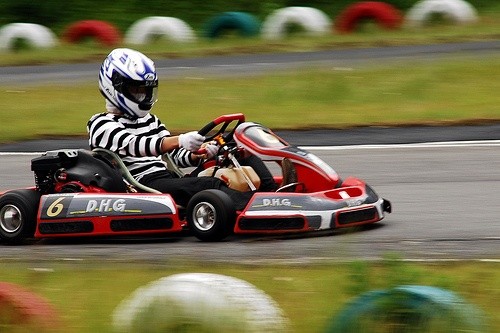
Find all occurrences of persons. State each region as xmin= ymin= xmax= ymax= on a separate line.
xmin=86 ymin=48 xmax=298 ymax=209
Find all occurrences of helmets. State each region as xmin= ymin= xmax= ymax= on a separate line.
xmin=98 ymin=48 xmax=159 ymax=121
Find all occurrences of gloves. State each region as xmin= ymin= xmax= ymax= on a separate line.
xmin=205 ymin=144 xmax=219 ymax=157
xmin=178 ymin=131 xmax=206 ymax=152
